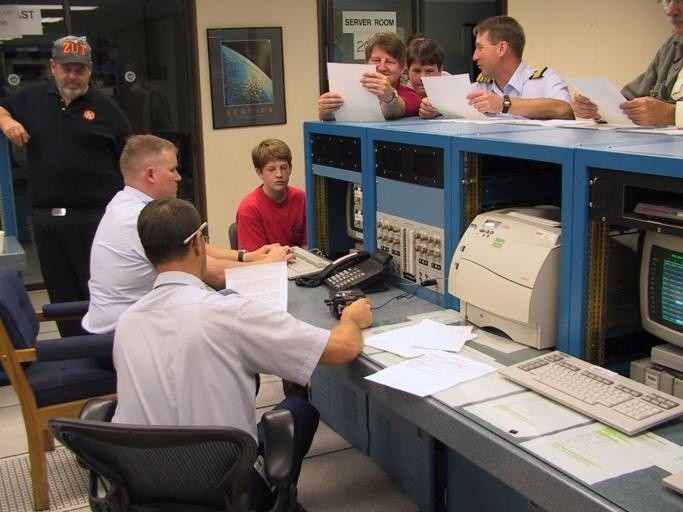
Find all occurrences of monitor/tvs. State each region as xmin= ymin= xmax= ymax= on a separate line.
xmin=346 ymin=183 xmax=363 ymax=241
xmin=639 ymin=229 xmax=682 ymax=373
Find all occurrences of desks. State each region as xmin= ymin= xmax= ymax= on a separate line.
xmin=289 ymin=241 xmax=682 ymax=512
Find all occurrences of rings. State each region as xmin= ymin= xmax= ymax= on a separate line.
xmin=374 ymin=84 xmax=377 ymax=88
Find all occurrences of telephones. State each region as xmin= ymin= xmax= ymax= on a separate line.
xmin=296 ymin=249 xmax=394 ymax=292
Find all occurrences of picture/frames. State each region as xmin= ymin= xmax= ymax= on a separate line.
xmin=206 ymin=25 xmax=286 ymax=129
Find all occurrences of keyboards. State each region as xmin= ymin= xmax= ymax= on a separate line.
xmin=287 ymin=246 xmax=332 ymax=280
xmin=498 ymin=350 xmax=682 ymax=438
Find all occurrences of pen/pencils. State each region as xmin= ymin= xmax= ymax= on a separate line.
xmin=184 ymin=222 xmax=208 ymax=244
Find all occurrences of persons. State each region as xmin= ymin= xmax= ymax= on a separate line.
xmin=112 ymin=197 xmax=375 ymax=512
xmin=235 ymin=139 xmax=309 ymax=253
xmin=79 ymin=134 xmax=299 ymax=399
xmin=317 ymin=32 xmax=422 ymax=120
xmin=419 ymin=16 xmax=575 ymax=122
xmin=0 ymin=35 xmax=139 ymax=339
xmin=401 ymin=36 xmax=454 ymax=96
xmin=571 ymin=0 xmax=683 ymax=129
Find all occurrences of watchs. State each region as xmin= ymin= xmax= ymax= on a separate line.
xmin=383 ymin=88 xmax=398 ymax=104
xmin=502 ymin=95 xmax=512 ymax=113
xmin=237 ymin=249 xmax=247 ymax=262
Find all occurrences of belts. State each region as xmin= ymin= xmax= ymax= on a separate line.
xmin=31 ymin=205 xmax=105 ymax=216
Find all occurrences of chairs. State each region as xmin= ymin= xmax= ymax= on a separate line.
xmin=47 ymin=417 xmax=282 ymax=509
xmin=0 ymin=262 xmax=118 ymax=511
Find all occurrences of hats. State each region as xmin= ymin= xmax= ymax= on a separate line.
xmin=52 ymin=36 xmax=92 ymax=67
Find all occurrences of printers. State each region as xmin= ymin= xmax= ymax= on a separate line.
xmin=447 ymin=202 xmax=561 ymax=349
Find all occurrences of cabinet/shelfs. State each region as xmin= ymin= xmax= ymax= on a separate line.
xmin=303 ymin=116 xmax=683 ymax=359
